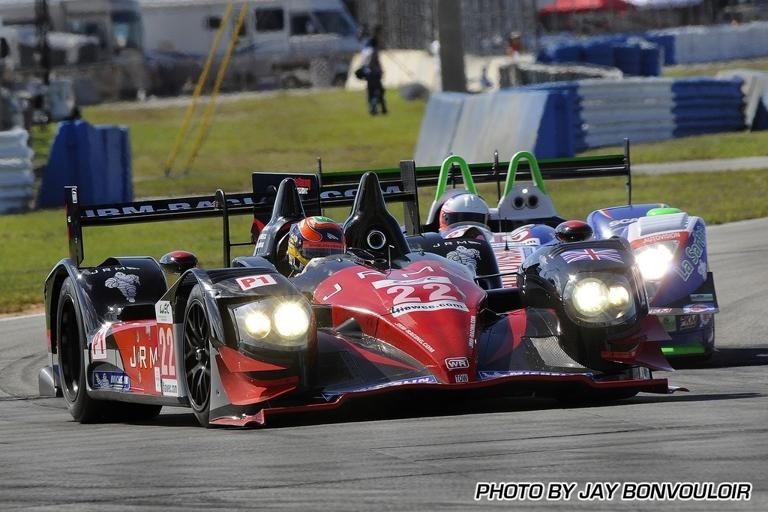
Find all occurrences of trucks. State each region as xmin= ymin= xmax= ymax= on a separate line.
xmin=0 ymin=1 xmax=363 ymax=88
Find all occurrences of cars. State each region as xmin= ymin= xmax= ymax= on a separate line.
xmin=33 ymin=158 xmax=692 ymax=430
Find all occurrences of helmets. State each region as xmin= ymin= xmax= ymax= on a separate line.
xmin=286 ymin=215 xmax=347 ymax=270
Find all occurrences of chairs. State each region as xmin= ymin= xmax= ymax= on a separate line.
xmin=238 ymin=172 xmax=413 ymax=272
xmin=417 ymin=151 xmax=570 ymax=233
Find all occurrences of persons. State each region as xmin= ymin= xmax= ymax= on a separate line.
xmin=283 ymin=214 xmax=346 ymax=278
xmin=353 ymin=24 xmax=390 ymax=116
